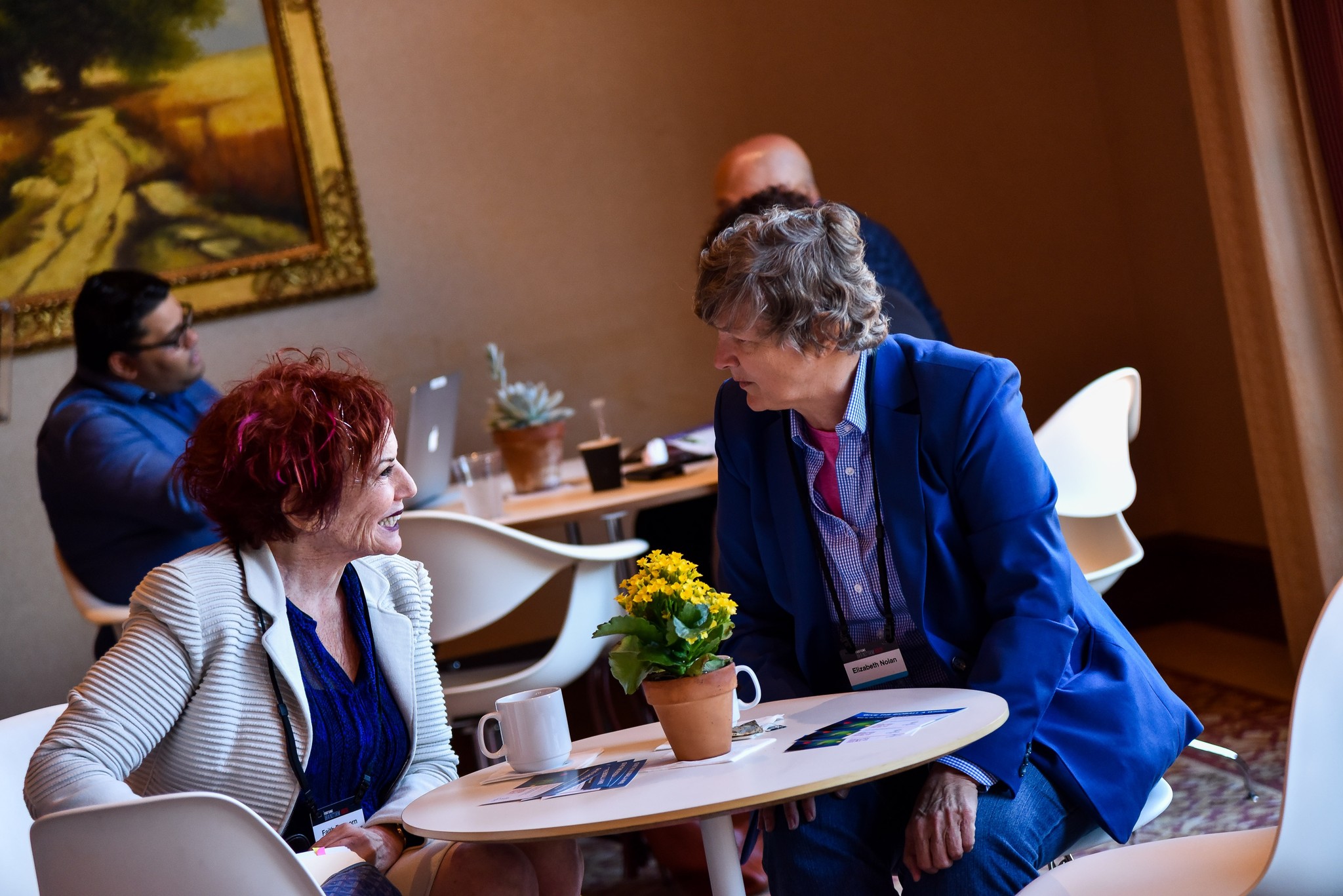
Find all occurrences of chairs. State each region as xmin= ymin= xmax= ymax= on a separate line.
xmin=1 ymin=509 xmax=650 ymax=896
xmin=1014 ymin=368 xmax=1343 ymax=896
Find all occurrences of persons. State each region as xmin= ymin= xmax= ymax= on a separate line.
xmin=692 ymin=200 xmax=1206 ymax=896
xmin=34 ymin=267 xmax=227 ymax=661
xmin=699 ymin=134 xmax=956 ymax=349
xmin=23 ymin=347 xmax=583 ymax=895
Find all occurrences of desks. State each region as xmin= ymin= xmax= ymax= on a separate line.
xmin=422 ymin=456 xmax=719 ymax=577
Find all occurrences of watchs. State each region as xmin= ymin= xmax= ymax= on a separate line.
xmin=392 ymin=823 xmax=409 ymax=846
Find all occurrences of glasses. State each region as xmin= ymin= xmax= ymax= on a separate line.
xmin=125 ymin=300 xmax=193 ymax=353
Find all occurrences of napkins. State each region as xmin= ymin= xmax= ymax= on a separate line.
xmin=639 ymin=714 xmax=786 ymax=773
xmin=480 ymin=748 xmax=603 ymax=788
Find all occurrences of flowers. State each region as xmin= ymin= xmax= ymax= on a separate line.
xmin=588 ymin=541 xmax=739 ymax=701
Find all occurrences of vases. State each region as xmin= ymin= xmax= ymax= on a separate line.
xmin=640 ymin=657 xmax=738 ymax=764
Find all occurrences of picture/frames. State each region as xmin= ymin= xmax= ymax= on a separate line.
xmin=0 ymin=0 xmax=379 ymax=358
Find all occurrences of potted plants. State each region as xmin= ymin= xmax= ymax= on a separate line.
xmin=481 ymin=340 xmax=579 ymax=493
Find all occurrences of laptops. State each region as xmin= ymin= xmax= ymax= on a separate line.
xmin=401 ymin=374 xmax=461 ymax=509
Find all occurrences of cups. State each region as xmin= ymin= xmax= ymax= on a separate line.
xmin=697 ymin=656 xmax=761 ymax=727
xmin=577 ymin=437 xmax=622 ymax=492
xmin=451 ymin=448 xmax=502 ymax=519
xmin=477 ymin=688 xmax=572 ymax=773
